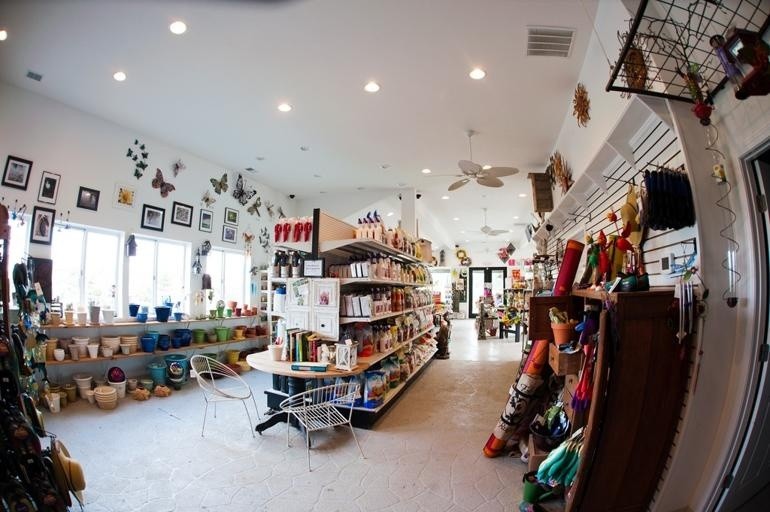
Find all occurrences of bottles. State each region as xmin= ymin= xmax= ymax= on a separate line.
xmin=391 ymin=286 xmax=406 ymax=311
xmin=394 ymin=232 xmax=416 ymax=257
xmin=389 ymin=324 xmax=422 ymax=344
xmin=391 ymin=262 xmax=414 ymax=283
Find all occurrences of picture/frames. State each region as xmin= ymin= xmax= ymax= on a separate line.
xmin=0 ymin=155 xmax=239 ymax=245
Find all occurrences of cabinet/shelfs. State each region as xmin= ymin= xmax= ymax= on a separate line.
xmin=497 ymin=288 xmax=533 ymax=341
xmin=529 ymin=289 xmax=697 ymax=512
xmin=246 ymin=208 xmax=438 ymax=430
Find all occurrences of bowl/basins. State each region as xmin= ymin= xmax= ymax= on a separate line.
xmin=33 ymin=302 xmax=267 ymax=413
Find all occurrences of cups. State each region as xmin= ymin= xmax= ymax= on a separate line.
xmin=266 ymin=345 xmax=286 ymax=362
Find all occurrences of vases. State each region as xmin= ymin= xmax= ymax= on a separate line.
xmin=30 ymin=301 xmax=270 ymax=410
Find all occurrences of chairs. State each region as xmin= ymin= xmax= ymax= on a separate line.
xmin=279 ymin=382 xmax=366 ymax=472
xmin=191 ymin=355 xmax=260 ymax=438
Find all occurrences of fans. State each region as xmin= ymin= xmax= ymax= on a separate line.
xmin=448 ymin=130 xmax=519 ymax=191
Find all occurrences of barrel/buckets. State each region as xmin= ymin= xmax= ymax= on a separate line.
xmin=550 ymin=320 xmax=579 ymax=346
xmin=31 ymin=300 xmax=268 ymax=414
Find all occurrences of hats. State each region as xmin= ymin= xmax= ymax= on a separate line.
xmin=621 ymin=183 xmax=643 ymax=246
xmin=52 ymin=440 xmax=85 ymax=508
